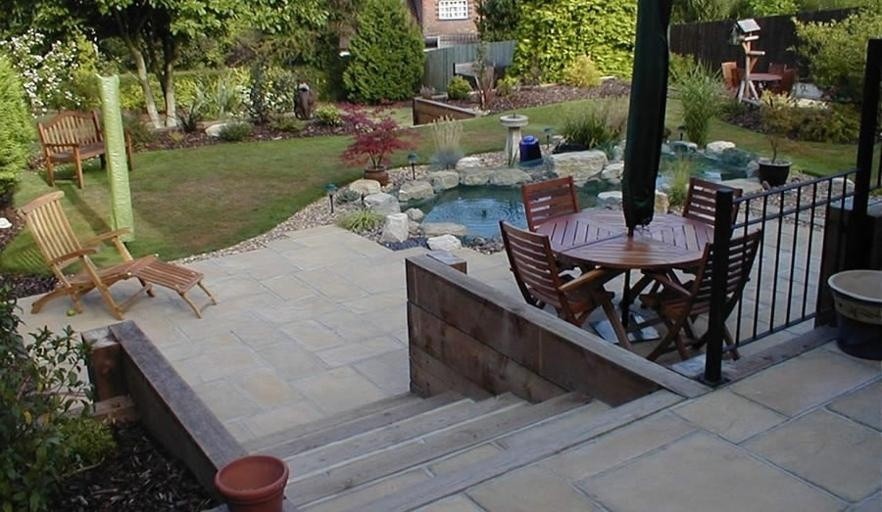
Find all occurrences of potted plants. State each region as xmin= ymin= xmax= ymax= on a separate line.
xmin=757 ymin=95 xmax=805 ymax=191
xmin=334 ymin=97 xmax=425 ymax=187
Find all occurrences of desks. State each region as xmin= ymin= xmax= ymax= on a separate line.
xmin=536 ymin=209 xmax=719 ymax=346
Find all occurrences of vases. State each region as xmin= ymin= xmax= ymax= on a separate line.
xmin=214 ymin=453 xmax=289 ymax=512
xmin=828 ymin=269 xmax=882 ymax=361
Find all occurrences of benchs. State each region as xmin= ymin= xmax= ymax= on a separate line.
xmin=37 ymin=109 xmax=136 ymax=189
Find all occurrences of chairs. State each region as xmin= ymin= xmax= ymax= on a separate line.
xmin=522 ymin=174 xmax=616 ymax=310
xmin=499 ymin=219 xmax=635 ymax=352
xmin=638 ymin=228 xmax=762 ymax=362
xmin=640 ymin=175 xmax=743 ymax=309
xmin=722 ymin=61 xmax=798 ymax=98
xmin=20 ymin=190 xmax=220 ymax=320
